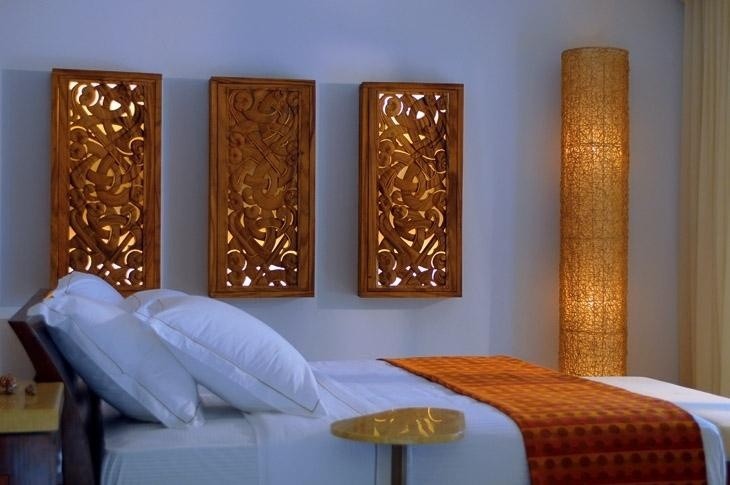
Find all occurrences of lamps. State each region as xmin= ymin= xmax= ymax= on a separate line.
xmin=558 ymin=46 xmax=631 ymax=382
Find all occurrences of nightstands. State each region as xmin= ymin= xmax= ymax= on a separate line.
xmin=0 ymin=378 xmax=67 ymax=485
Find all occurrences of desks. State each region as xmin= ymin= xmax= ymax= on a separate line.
xmin=331 ymin=407 xmax=465 ymax=485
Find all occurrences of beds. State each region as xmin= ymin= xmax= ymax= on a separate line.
xmin=6 ymin=288 xmax=729 ymax=484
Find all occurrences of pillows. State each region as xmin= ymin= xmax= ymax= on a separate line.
xmin=117 ymin=289 xmax=330 ymax=422
xmin=25 ymin=270 xmax=205 ymax=432
